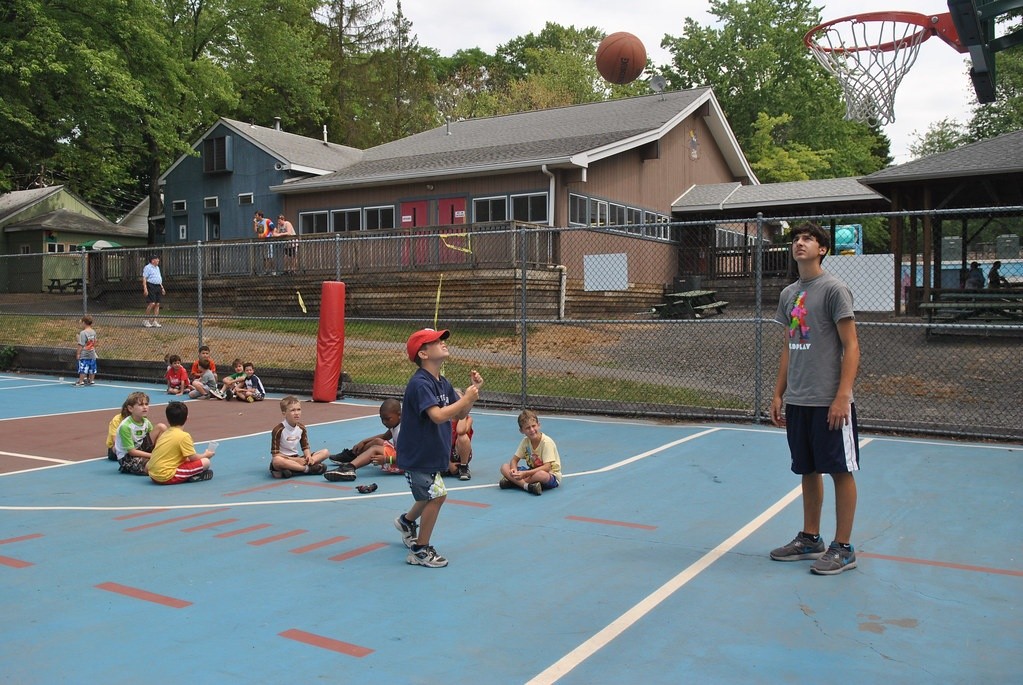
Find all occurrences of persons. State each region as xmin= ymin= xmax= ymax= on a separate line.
xmin=499 ymin=410 xmax=562 ymax=496
xmin=440 ymin=387 xmax=473 ymax=480
xmin=106 ymin=400 xmax=132 ymax=462
xmin=140 ymin=254 xmax=165 ymax=328
xmin=164 ymin=346 xmax=266 ymax=403
xmin=768 ymin=220 xmax=860 ymax=575
xmin=146 ymin=401 xmax=215 ymax=485
xmin=269 ymin=396 xmax=330 ymax=479
xmin=967 ymin=262 xmax=984 ymax=290
xmin=272 ymin=214 xmax=298 ymax=275
xmin=115 ymin=391 xmax=168 ymax=476
xmin=987 ymin=261 xmax=1005 ymax=290
xmin=323 ymin=398 xmax=406 ymax=482
xmin=394 ymin=328 xmax=483 ymax=569
xmin=70 ymin=315 xmax=97 ymax=387
xmin=252 ymin=209 xmax=277 ymax=275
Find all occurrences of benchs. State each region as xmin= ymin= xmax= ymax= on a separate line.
xmin=45 ymin=283 xmax=84 ymax=289
xmin=693 ymin=300 xmax=730 ymax=310
xmin=922 ymin=315 xmax=1023 ymax=340
xmin=650 ymin=299 xmax=684 ymax=308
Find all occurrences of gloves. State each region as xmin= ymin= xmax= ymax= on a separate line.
xmin=356 ymin=483 xmax=378 ymax=493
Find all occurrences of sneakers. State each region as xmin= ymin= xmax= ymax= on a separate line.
xmin=528 ymin=482 xmax=542 ymax=495
xmin=305 ymin=463 xmax=327 ymax=476
xmin=407 ymin=543 xmax=448 ymax=567
xmin=226 ymin=388 xmax=234 ymax=402
xmin=142 ymin=320 xmax=161 ymax=327
xmin=272 ymin=470 xmax=294 ymax=478
xmin=395 ymin=513 xmax=420 ymax=548
xmin=499 ymin=477 xmax=516 ymax=489
xmin=236 ymin=396 xmax=243 ymax=402
xmin=810 ymin=541 xmax=857 ymax=574
xmin=459 ymin=463 xmax=471 ymax=479
xmin=324 ymin=464 xmax=356 ymax=482
xmin=197 ymin=394 xmax=210 ymax=400
xmin=771 ymin=531 xmax=827 ymax=561
xmin=328 ymin=448 xmax=357 ymax=464
xmin=247 ymin=396 xmax=253 ymax=403
xmin=210 ymin=388 xmax=224 ymax=399
xmin=188 ymin=470 xmax=214 ymax=482
xmin=76 ymin=380 xmax=95 ymax=388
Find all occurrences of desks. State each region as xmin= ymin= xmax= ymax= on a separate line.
xmin=662 ymin=290 xmax=723 ymax=320
xmin=939 ymin=292 xmax=1023 ymax=301
xmin=47 ymin=278 xmax=84 ymax=294
xmin=919 ymin=301 xmax=1023 ymax=318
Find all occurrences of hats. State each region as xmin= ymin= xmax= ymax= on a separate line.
xmin=406 ymin=328 xmax=450 ymax=361
xmin=149 ymin=255 xmax=158 ymax=259
xmin=971 ymin=262 xmax=981 ymax=267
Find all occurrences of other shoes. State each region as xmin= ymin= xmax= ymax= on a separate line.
xmin=256 ymin=271 xmax=277 ymax=276
xmin=183 ymin=389 xmax=190 ymax=395
xmin=290 ymin=269 xmax=296 ymax=276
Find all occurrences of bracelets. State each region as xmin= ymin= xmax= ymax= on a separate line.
xmin=390 ymin=456 xmax=392 ymax=465
xmin=384 ymin=455 xmax=387 ymax=464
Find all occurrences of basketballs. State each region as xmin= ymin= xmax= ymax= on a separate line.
xmin=596 ymin=31 xmax=648 ymax=85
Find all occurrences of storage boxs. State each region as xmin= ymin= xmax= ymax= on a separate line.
xmin=674 ymin=277 xmax=701 ymax=290
